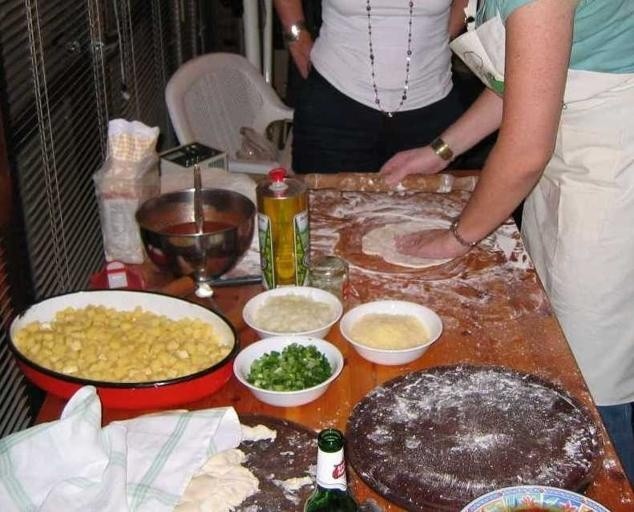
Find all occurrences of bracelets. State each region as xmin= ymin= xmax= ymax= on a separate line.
xmin=428 ymin=135 xmax=454 ymax=160
xmin=282 ymin=21 xmax=308 ymax=42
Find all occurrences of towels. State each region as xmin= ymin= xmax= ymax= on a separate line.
xmin=1 ymin=381 xmax=244 ymax=512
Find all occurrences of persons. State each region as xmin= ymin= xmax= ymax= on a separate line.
xmin=275 ymin=0 xmax=467 ymax=173
xmin=375 ymin=0 xmax=634 ymax=485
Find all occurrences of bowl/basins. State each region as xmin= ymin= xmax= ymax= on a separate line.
xmin=234 ymin=288 xmax=444 ymax=410
xmin=133 ymin=188 xmax=257 ymax=278
xmin=9 ymin=289 xmax=239 ymax=412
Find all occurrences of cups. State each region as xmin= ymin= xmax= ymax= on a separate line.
xmin=312 ymin=257 xmax=350 ymax=314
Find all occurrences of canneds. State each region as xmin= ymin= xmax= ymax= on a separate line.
xmin=308 ymin=256 xmax=349 ymax=310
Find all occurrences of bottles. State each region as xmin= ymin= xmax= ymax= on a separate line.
xmin=302 ymin=429 xmax=361 ymax=512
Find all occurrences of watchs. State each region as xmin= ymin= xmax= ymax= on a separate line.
xmin=448 ymin=219 xmax=478 ymax=247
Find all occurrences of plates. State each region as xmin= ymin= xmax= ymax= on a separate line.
xmin=460 ymin=484 xmax=611 ymax=512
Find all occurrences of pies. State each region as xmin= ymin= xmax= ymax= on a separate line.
xmin=362 ymin=219 xmax=454 ymax=269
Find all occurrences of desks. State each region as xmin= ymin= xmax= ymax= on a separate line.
xmin=23 ymin=154 xmax=634 ymax=512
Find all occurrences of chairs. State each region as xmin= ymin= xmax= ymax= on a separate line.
xmin=162 ymin=48 xmax=300 ymax=174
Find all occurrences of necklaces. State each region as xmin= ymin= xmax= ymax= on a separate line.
xmin=368 ymin=1 xmax=414 ymax=119
